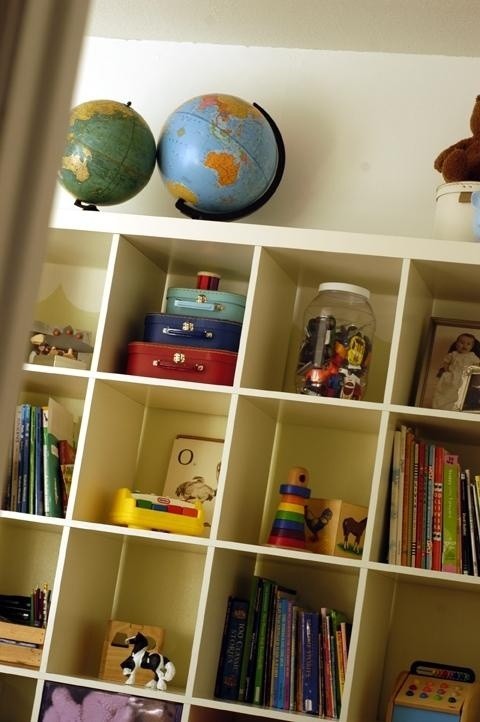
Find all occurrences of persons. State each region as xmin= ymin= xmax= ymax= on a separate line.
xmin=431 ymin=333 xmax=480 ymax=412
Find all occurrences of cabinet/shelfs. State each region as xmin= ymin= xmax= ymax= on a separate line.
xmin=0 ymin=203 xmax=480 ymax=722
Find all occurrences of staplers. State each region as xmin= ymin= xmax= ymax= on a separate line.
xmin=0 ymin=594 xmax=31 ymax=621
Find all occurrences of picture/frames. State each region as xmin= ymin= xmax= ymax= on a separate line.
xmin=407 ymin=315 xmax=480 ymax=413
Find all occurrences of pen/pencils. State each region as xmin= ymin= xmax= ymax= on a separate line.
xmin=32 ymin=582 xmax=51 ymax=629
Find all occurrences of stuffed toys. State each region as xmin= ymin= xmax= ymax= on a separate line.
xmin=434 ymin=94 xmax=480 ymax=184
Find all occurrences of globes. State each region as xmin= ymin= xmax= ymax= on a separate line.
xmin=55 ymin=100 xmax=156 ymax=211
xmin=158 ymin=94 xmax=286 ymax=223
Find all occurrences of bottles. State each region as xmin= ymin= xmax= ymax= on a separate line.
xmin=294 ymin=282 xmax=377 ymax=400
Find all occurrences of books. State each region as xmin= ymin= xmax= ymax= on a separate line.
xmin=383 ymin=424 xmax=480 ymax=577
xmin=211 ymin=574 xmax=349 ymax=720
xmin=3 ymin=397 xmax=83 ymax=518
